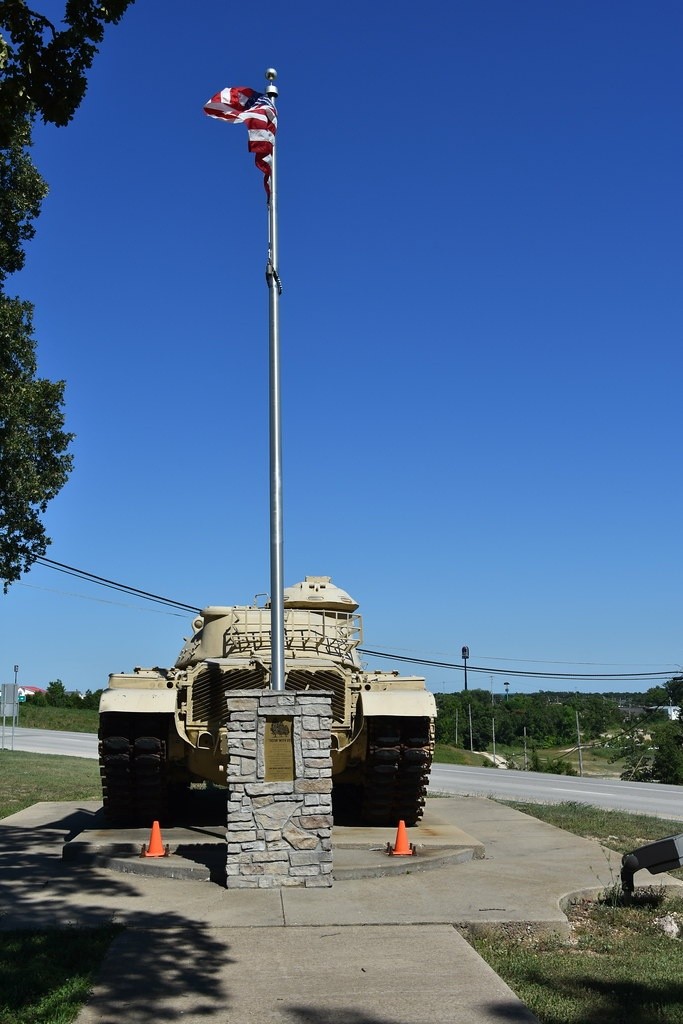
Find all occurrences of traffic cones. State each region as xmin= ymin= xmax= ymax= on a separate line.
xmin=143 ymin=820 xmax=172 ymax=859
xmin=387 ymin=820 xmax=416 ymax=856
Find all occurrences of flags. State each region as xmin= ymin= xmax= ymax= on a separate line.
xmin=203 ymin=87 xmax=277 ymax=197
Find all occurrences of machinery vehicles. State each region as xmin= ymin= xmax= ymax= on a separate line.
xmin=97 ymin=574 xmax=441 ymax=825
